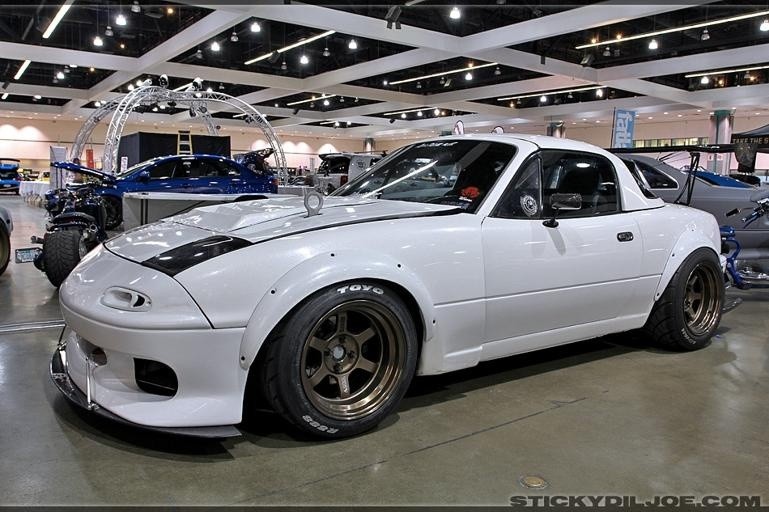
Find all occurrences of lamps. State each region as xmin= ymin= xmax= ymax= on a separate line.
xmin=648 ymin=7 xmax=769 ymax=50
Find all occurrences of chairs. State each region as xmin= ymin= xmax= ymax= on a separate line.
xmin=462 ymin=145 xmax=608 ymax=215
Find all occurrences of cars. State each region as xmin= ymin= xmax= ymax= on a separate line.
xmin=0 ymin=129 xmax=769 ymax=448
